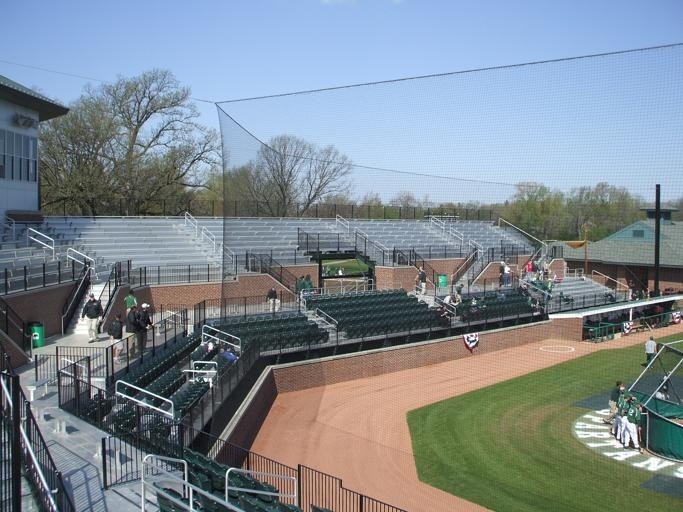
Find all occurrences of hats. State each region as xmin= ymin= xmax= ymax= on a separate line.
xmin=141 ymin=303 xmax=150 ymax=308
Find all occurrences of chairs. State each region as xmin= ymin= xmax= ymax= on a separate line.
xmin=116 ymin=426 xmax=331 ymax=512
xmin=70 ymin=333 xmax=237 ymax=431
xmin=202 ymin=310 xmax=329 ymax=352
xmin=301 ymin=288 xmax=452 ymax=340
xmin=434 ymin=287 xmax=540 ymax=324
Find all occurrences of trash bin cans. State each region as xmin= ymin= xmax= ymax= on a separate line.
xmin=28 ymin=322 xmax=45 ymax=348
xmin=437 ymin=274 xmax=447 ymax=287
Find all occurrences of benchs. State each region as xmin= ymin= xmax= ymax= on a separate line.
xmin=43 ymin=407 xmax=70 ymax=434
xmin=0 ymin=215 xmax=537 ymax=297
xmin=26 ymin=378 xmax=50 ymax=402
xmin=518 ymin=275 xmax=623 ymax=313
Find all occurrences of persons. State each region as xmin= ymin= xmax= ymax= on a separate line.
xmin=136 ymin=303 xmax=154 ymax=352
xmin=454 ymin=283 xmax=464 ymax=302
xmin=123 ymin=289 xmax=137 ymax=316
xmin=443 ymin=294 xmax=457 ymax=306
xmin=496 ymin=258 xmax=559 ymax=297
xmin=264 ymin=285 xmax=281 ymax=311
xmin=125 ymin=305 xmax=138 ymax=361
xmin=80 ymin=293 xmax=104 ymax=342
xmin=644 ymin=335 xmax=657 ymax=369
xmin=107 ymin=312 xmax=122 ymax=366
xmin=602 ymin=380 xmax=643 ymax=452
xmin=414 ymin=267 xmax=427 ymax=295
xmin=298 ymin=273 xmax=312 ymax=292
